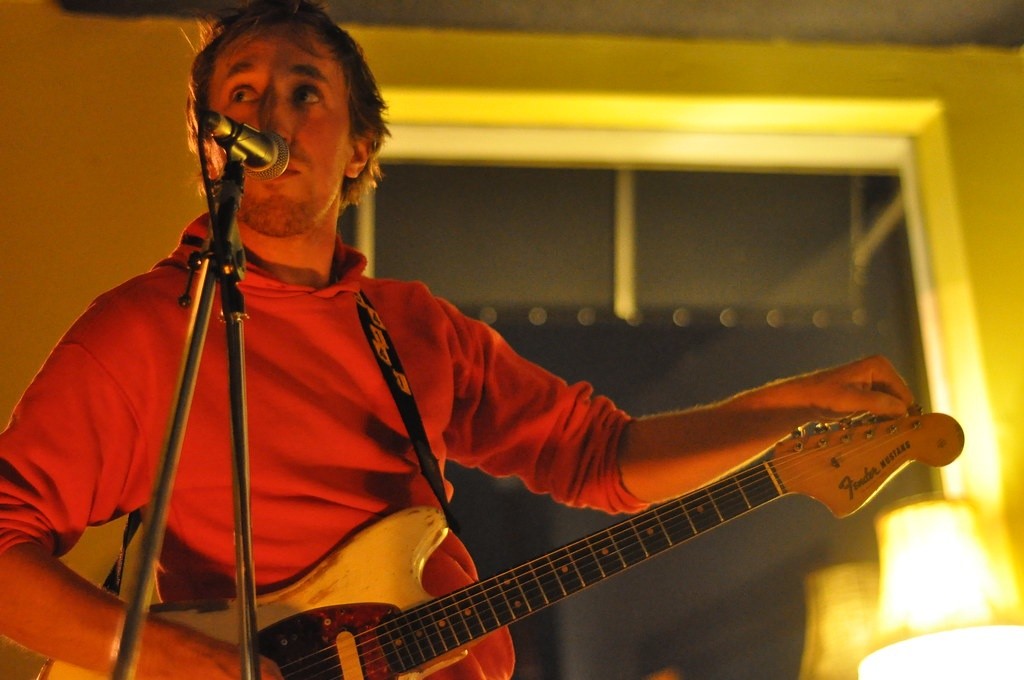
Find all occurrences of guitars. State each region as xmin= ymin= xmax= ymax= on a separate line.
xmin=26 ymin=398 xmax=966 ymax=680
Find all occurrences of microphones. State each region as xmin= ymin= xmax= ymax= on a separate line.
xmin=196 ymin=111 xmax=291 ymax=180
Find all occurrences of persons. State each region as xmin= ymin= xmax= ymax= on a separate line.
xmin=0 ymin=1 xmax=921 ymax=680
